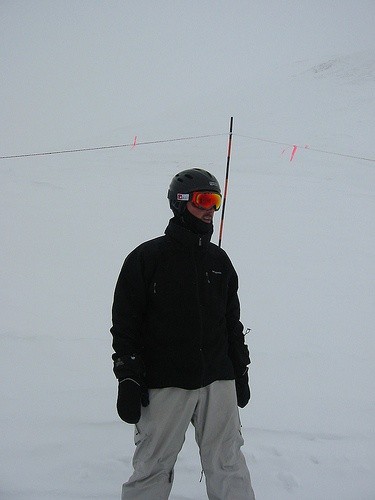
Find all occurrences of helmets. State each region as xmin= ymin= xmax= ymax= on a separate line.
xmin=167 ymin=168 xmax=222 ymax=201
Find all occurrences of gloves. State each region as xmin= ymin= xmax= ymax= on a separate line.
xmin=112 ymin=353 xmax=149 ymax=424
xmin=235 ymin=365 xmax=250 ymax=408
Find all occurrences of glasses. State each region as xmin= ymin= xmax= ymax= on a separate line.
xmin=188 ymin=191 xmax=223 ymax=212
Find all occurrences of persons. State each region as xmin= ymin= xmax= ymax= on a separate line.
xmin=110 ymin=168 xmax=256 ymax=500
